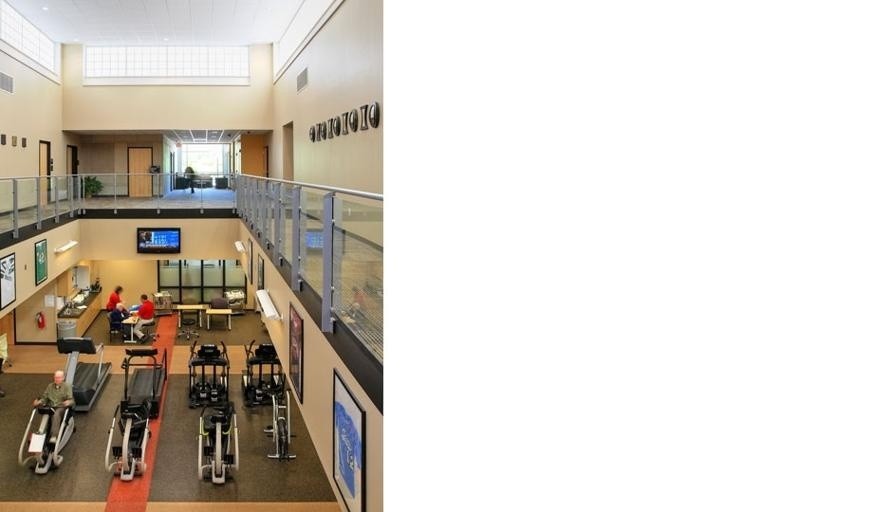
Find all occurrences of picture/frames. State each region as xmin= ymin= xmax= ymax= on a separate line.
xmin=1 ymin=252 xmax=18 ymax=309
xmin=245 ymin=238 xmax=267 ymax=293
xmin=32 ymin=237 xmax=49 ymax=285
xmin=327 ymin=367 xmax=371 ymax=512
xmin=285 ymin=302 xmax=307 ymax=404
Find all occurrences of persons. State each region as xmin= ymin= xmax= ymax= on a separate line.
xmin=139 ymin=232 xmax=152 ymax=246
xmin=111 ymin=302 xmax=130 ymax=339
xmin=32 ymin=370 xmax=76 ymax=444
xmin=107 ymin=286 xmax=122 ymax=311
xmin=133 ymin=294 xmax=154 ymax=342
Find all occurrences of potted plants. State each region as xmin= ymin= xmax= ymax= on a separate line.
xmin=83 ymin=174 xmax=102 ymax=200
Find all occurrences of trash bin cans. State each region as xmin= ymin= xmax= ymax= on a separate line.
xmin=58 ymin=320 xmax=76 ymax=337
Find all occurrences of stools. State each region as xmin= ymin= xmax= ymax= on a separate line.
xmin=178 ymin=318 xmax=199 ymax=341
xmin=138 ymin=319 xmax=156 ymax=340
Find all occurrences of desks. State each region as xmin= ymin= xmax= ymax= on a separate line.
xmin=123 ymin=308 xmax=142 ymax=344
xmin=178 ymin=299 xmax=232 ymax=332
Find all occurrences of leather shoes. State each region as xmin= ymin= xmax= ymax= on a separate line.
xmin=124 ymin=337 xmax=130 ymax=340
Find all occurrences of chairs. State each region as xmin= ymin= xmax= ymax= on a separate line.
xmin=104 ymin=309 xmax=123 ymax=345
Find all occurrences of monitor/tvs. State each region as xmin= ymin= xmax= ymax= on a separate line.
xmin=300 ymin=229 xmax=345 ymax=256
xmin=137 ymin=227 xmax=180 ymax=254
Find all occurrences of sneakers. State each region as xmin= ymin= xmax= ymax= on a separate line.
xmin=141 ymin=335 xmax=149 ymax=344
xmin=48 ymin=436 xmax=56 ymax=444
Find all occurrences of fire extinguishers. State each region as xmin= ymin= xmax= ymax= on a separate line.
xmin=36 ymin=311 xmax=44 ymax=328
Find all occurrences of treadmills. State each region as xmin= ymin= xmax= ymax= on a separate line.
xmin=120 ymin=348 xmax=167 ymax=419
xmin=57 ymin=336 xmax=111 ymax=412
